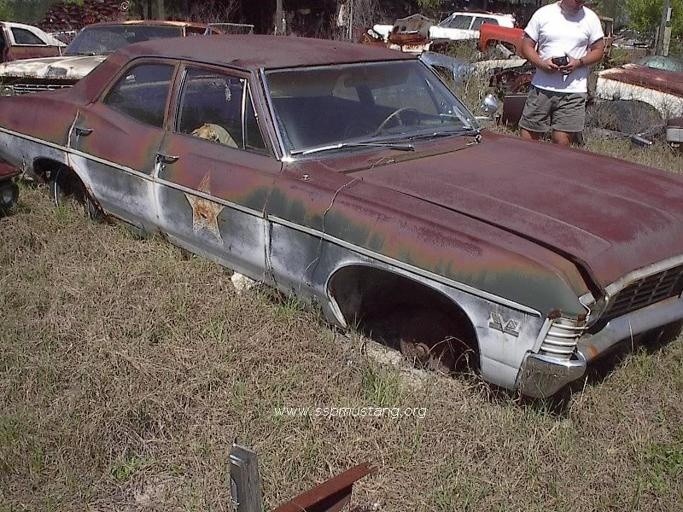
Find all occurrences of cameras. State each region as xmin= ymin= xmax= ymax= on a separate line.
xmin=552 ymin=56 xmax=568 ymax=65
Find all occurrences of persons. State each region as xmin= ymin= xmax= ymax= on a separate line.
xmin=519 ymin=0 xmax=605 ymax=147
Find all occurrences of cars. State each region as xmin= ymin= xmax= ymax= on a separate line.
xmin=0 ymin=21 xmax=231 ymax=98
xmin=0 ymin=31 xmax=682 ymax=407
xmin=369 ymin=10 xmax=518 ymax=48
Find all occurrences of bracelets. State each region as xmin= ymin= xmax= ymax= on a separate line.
xmin=580 ymin=57 xmax=583 ymax=66
